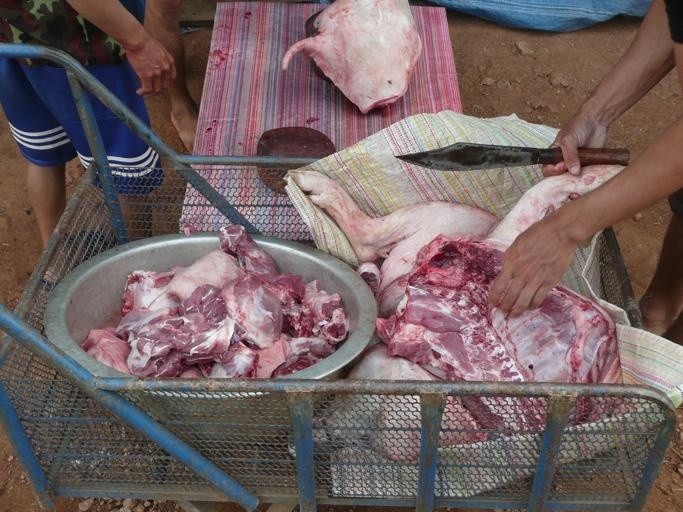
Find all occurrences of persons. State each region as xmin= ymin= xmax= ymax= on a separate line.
xmin=0 ymin=0 xmax=179 ymax=286
xmin=144 ymin=0 xmax=203 ymax=156
xmin=487 ymin=1 xmax=683 ymax=350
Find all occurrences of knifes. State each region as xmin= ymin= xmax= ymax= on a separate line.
xmin=395 ymin=139 xmax=630 ymax=172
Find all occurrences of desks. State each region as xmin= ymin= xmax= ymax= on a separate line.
xmin=179 ymin=1 xmax=463 ymax=248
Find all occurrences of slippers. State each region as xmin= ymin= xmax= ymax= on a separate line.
xmin=41 ymin=232 xmax=111 ymax=291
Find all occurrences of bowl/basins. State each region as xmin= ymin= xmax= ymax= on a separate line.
xmin=42 ymin=230 xmax=377 ymax=438
